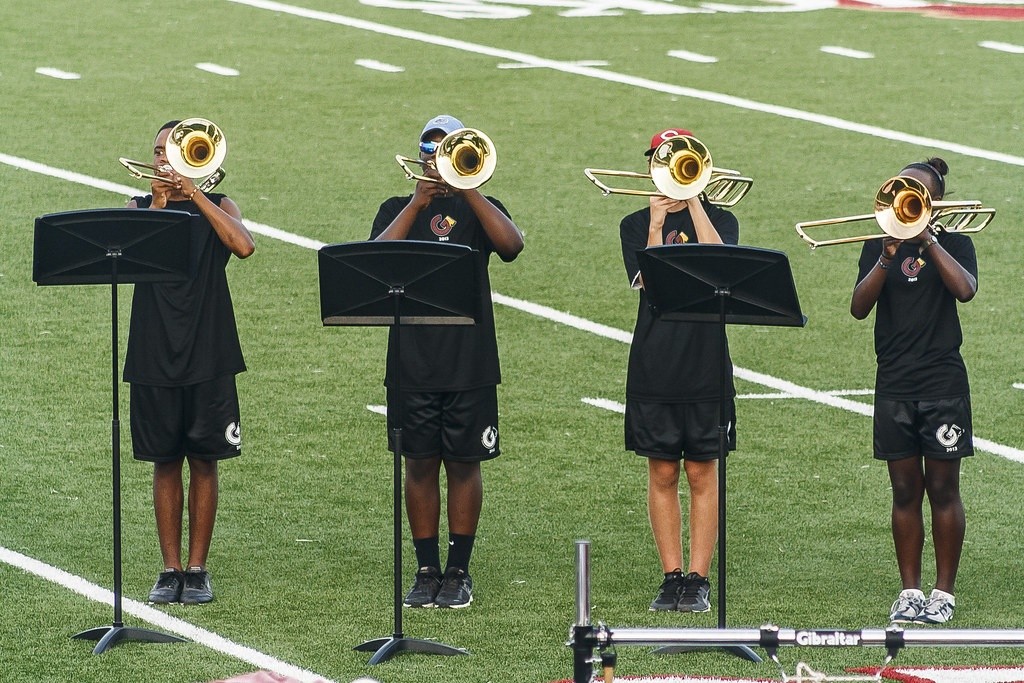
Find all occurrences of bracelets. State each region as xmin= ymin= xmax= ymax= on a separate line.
xmin=877 ymin=252 xmax=896 ymax=270
xmin=188 ymin=185 xmax=200 ymax=200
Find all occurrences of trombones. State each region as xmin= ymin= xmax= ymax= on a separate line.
xmin=395 ymin=127 xmax=498 ymax=192
xmin=117 ymin=117 xmax=227 ymax=200
xmin=793 ymin=174 xmax=997 ymax=251
xmin=582 ymin=134 xmax=754 ymax=209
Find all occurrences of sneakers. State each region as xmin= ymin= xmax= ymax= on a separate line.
xmin=403 ymin=566 xmax=473 ymax=608
xmin=890 ymin=588 xmax=956 ymax=625
xmin=650 ymin=567 xmax=712 ymax=613
xmin=148 ymin=565 xmax=213 ymax=606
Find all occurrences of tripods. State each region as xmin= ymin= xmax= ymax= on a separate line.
xmin=33 ymin=208 xmax=192 ymax=654
xmin=318 ymin=241 xmax=483 ymax=667
xmin=636 ymin=244 xmax=808 ymax=661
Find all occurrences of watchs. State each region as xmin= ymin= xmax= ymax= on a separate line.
xmin=918 ymin=235 xmax=938 ymax=256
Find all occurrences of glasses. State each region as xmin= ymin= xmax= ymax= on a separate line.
xmin=418 ymin=140 xmax=440 ymax=155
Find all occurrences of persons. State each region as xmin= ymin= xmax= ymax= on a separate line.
xmin=364 ymin=112 xmax=524 ymax=611
xmin=121 ymin=119 xmax=259 ymax=606
xmin=618 ymin=130 xmax=741 ymax=613
xmin=845 ymin=154 xmax=980 ymax=626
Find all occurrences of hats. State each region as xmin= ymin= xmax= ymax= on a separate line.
xmin=644 ymin=128 xmax=693 ymax=157
xmin=419 ymin=115 xmax=465 ymax=142
